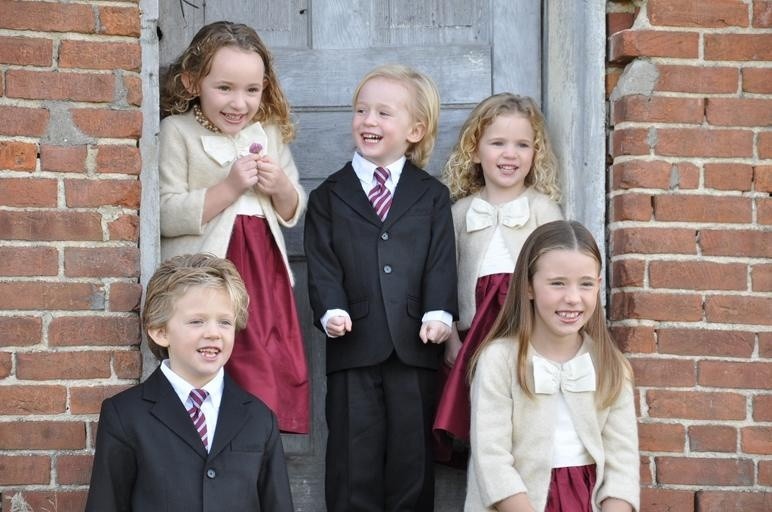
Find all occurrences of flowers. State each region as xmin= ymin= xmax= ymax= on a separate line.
xmin=249 ymin=142 xmax=263 ymax=155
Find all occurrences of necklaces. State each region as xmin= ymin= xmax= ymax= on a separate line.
xmin=193 ymin=105 xmax=222 ymax=138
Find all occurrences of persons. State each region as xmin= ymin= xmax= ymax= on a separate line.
xmin=83 ymin=252 xmax=295 ymax=510
xmin=159 ymin=20 xmax=309 ymax=435
xmin=440 ymin=92 xmax=566 ymax=511
xmin=304 ymin=62 xmax=456 ymax=511
xmin=457 ymin=220 xmax=641 ymax=512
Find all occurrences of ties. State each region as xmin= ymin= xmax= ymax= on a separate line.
xmin=368 ymin=166 xmax=393 ymax=223
xmin=187 ymin=390 xmax=209 ymax=455
